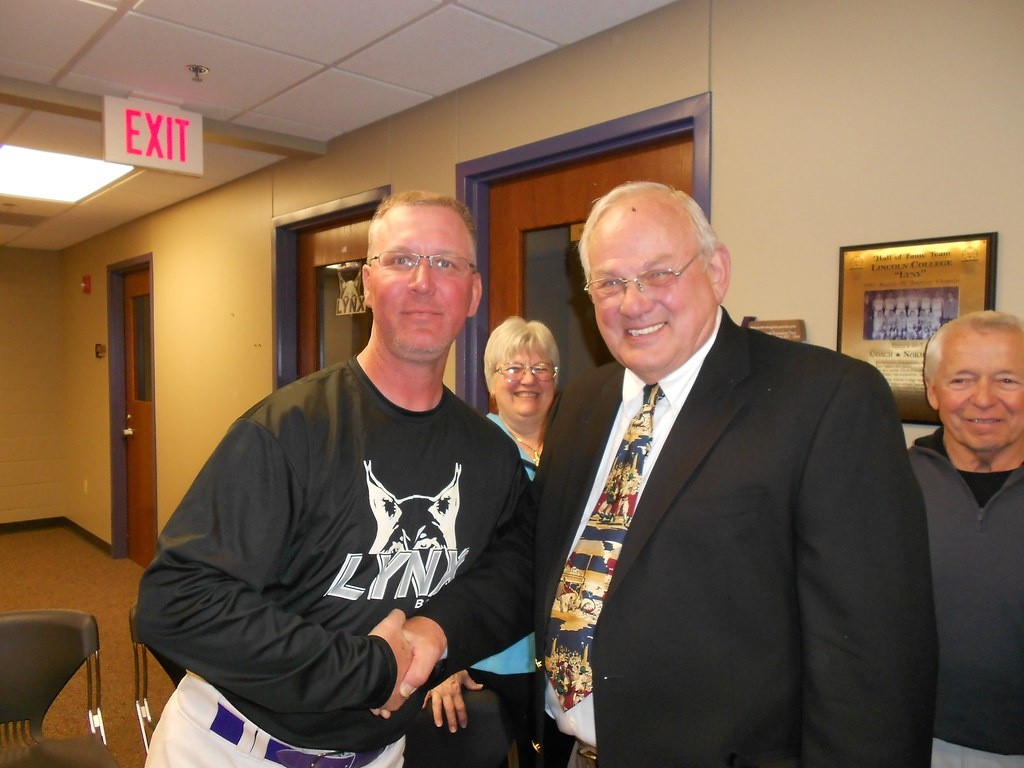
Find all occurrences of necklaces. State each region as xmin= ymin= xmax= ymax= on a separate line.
xmin=510 ymin=431 xmax=544 ymax=467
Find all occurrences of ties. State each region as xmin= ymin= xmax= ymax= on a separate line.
xmin=539 ymin=383 xmax=666 ymax=714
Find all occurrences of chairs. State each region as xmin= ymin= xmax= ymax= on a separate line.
xmin=0 ymin=608 xmax=120 ymax=768
xmin=129 ymin=602 xmax=188 ymax=755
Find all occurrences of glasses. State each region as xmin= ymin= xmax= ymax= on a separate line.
xmin=368 ymin=250 xmax=478 ymax=279
xmin=494 ymin=361 xmax=558 ymax=383
xmin=584 ymin=249 xmax=705 ymax=309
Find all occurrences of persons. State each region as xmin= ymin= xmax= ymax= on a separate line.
xmin=139 ymin=188 xmax=533 ymax=768
xmin=908 ymin=310 xmax=1024 ymax=768
xmin=865 ymin=291 xmax=958 ymax=340
xmin=370 ymin=181 xmax=938 ymax=768
xmin=405 ymin=314 xmax=576 ymax=767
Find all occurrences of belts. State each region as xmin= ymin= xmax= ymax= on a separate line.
xmin=207 ymin=700 xmax=387 ymax=768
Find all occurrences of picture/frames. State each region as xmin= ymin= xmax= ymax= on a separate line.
xmin=836 ymin=231 xmax=999 ymax=426
